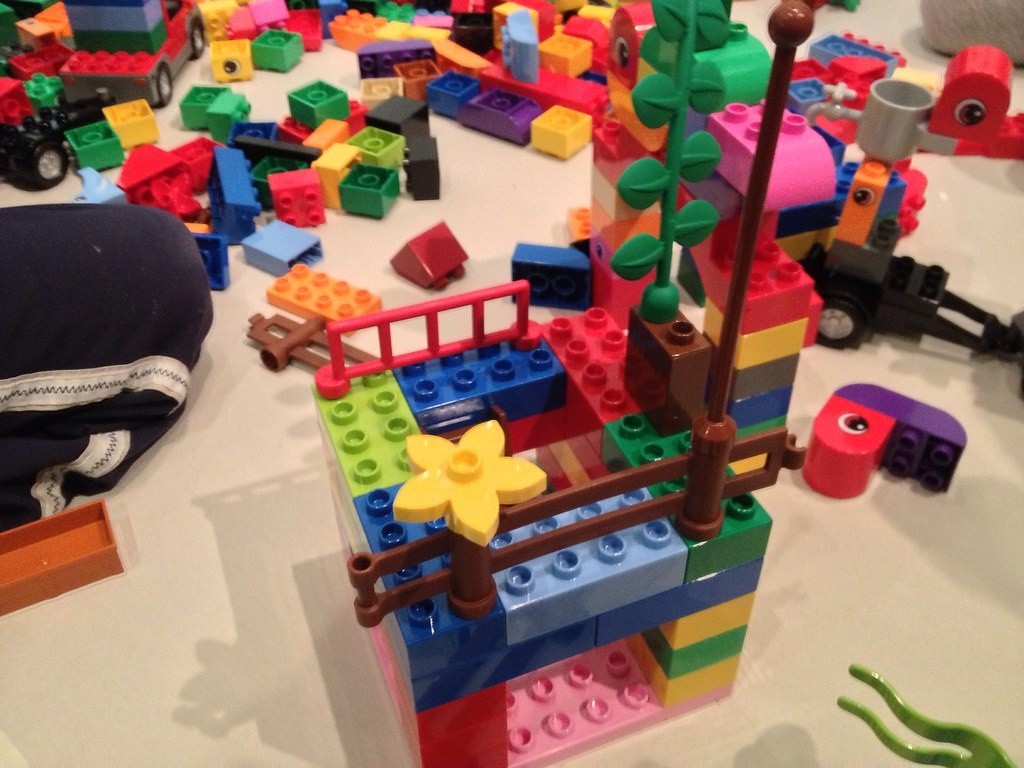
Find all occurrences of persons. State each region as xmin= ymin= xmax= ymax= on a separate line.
xmin=0 ymin=202 xmax=212 ymax=537
